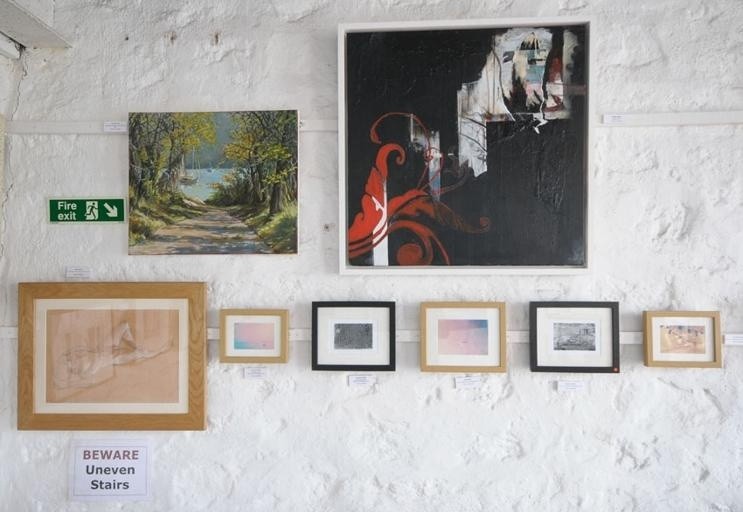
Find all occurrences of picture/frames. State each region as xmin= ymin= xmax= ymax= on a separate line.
xmin=336 ymin=16 xmax=600 ymax=278
xmin=641 ymin=309 xmax=725 ymax=369
xmin=309 ymin=298 xmax=399 ymax=374
xmin=527 ymin=300 xmax=623 ymax=375
xmin=218 ymin=306 xmax=289 ymax=365
xmin=15 ymin=281 xmax=209 ymax=431
xmin=417 ymin=298 xmax=509 ymax=376
